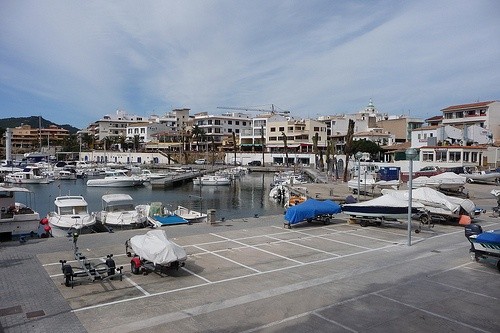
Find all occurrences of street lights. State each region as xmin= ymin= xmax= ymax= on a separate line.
xmin=355 ymin=153 xmax=363 ymax=204
xmin=404 ymin=149 xmax=419 ymax=247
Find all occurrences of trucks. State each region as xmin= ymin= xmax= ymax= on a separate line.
xmin=348 ymin=162 xmax=402 ymax=196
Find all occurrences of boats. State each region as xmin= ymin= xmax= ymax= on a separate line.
xmin=105 ymin=175 xmax=148 ymax=184
xmin=285 ymin=196 xmax=306 ymax=211
xmin=43 ymin=188 xmax=96 ymax=238
xmin=193 ymin=166 xmax=249 ymax=185
xmin=0 ymin=174 xmax=40 ymax=236
xmin=87 ymin=175 xmax=139 ymax=187
xmin=3 ymin=163 xmax=135 ymax=183
xmin=459 ymin=166 xmax=500 ymax=185
xmin=469 ymin=228 xmax=500 ymax=257
xmin=94 ymin=194 xmax=148 ymax=229
xmin=173 ymin=205 xmax=208 ymax=222
xmin=115 ymin=169 xmax=167 ymax=178
xmin=269 ymin=168 xmax=307 ymax=199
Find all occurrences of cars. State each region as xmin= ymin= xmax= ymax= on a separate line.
xmin=415 ymin=165 xmax=444 ymax=177
